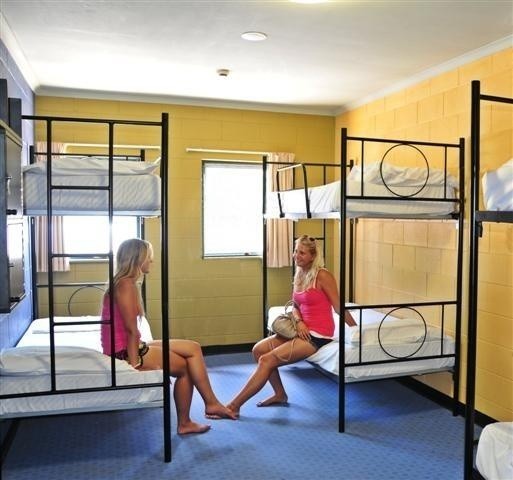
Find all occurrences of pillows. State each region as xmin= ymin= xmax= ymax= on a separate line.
xmin=0 ymin=346 xmax=141 ymax=376
xmin=24 ymin=157 xmax=161 ymax=175
xmin=333 ymin=318 xmax=453 ymax=347
xmin=346 ymin=162 xmax=458 ymax=188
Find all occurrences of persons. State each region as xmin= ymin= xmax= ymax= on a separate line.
xmin=205 ymin=235 xmax=357 ymax=420
xmin=100 ymin=239 xmax=237 ymax=435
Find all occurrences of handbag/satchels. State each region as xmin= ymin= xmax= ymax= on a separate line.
xmin=273 ymin=316 xmax=298 ymax=339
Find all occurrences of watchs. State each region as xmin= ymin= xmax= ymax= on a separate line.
xmin=295 ymin=319 xmax=303 ymax=325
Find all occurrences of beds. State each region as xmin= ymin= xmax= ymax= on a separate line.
xmin=0 ymin=113 xmax=171 ymax=463
xmin=263 ymin=128 xmax=465 ymax=433
xmin=465 ymin=81 xmax=512 ymax=479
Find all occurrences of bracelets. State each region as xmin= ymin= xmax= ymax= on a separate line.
xmin=133 ymin=363 xmax=141 ymax=368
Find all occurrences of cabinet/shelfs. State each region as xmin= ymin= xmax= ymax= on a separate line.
xmin=0 ymin=119 xmax=25 ymax=314
